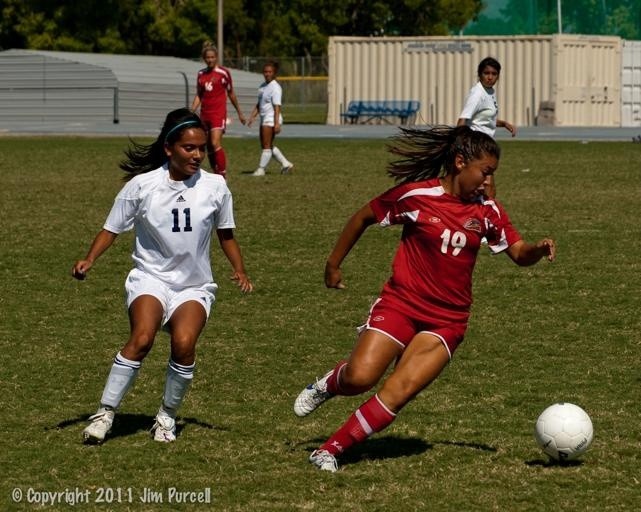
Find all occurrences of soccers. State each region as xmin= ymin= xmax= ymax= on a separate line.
xmin=534 ymin=403 xmax=593 ymax=463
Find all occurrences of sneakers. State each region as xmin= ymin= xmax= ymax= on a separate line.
xmin=309 ymin=450 xmax=339 ymax=473
xmin=253 ymin=168 xmax=265 ymax=176
xmin=293 ymin=369 xmax=335 ymax=417
xmin=152 ymin=406 xmax=177 ymax=442
xmin=281 ymin=163 xmax=294 ymax=175
xmin=83 ymin=407 xmax=115 ymax=444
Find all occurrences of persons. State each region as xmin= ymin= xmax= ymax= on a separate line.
xmin=292 ymin=124 xmax=557 ymax=474
xmin=247 ymin=60 xmax=294 ymax=177
xmin=456 ymin=58 xmax=517 ymax=201
xmin=189 ymin=40 xmax=249 ymax=181
xmin=71 ymin=109 xmax=255 ymax=446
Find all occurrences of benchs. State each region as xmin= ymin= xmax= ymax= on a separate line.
xmin=339 ymin=100 xmax=420 ymax=125
